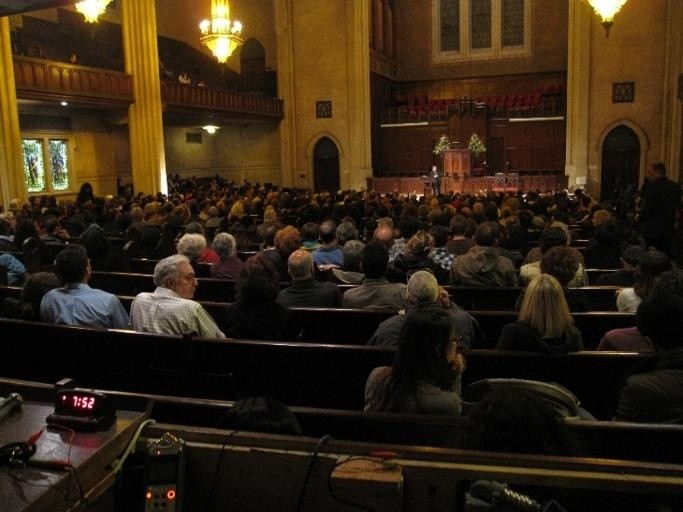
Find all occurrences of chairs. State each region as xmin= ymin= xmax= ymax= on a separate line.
xmin=398 ymin=82 xmax=563 ymax=122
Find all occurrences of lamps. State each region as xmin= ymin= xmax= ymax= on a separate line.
xmin=72 ymin=0 xmax=111 ymax=24
xmin=202 ymin=118 xmax=221 ymax=134
xmin=197 ymin=0 xmax=246 ymax=65
xmin=582 ymin=0 xmax=629 ymax=39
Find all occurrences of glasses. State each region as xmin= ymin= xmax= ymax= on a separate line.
xmin=448 ymin=336 xmax=463 ymax=347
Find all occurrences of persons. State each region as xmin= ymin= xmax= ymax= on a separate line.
xmin=358 ymin=308 xmax=683 ymax=511
xmin=215 ymin=394 xmax=304 ymax=436
xmin=0 ymin=162 xmax=683 ymax=345
